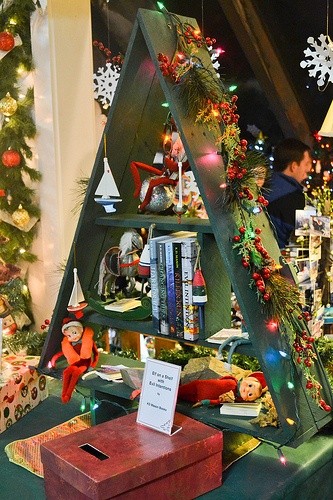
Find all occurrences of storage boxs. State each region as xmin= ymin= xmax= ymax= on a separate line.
xmin=40 ymin=412 xmax=223 ymax=500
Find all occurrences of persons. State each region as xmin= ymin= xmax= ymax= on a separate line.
xmin=261 ymin=138 xmax=311 ymax=311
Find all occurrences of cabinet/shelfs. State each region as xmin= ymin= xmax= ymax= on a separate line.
xmin=37 ymin=7 xmax=333 ymax=448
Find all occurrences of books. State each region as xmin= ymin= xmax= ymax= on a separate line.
xmin=148 ymin=232 xmax=203 ymax=341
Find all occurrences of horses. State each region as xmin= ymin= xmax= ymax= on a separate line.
xmin=98 ymin=229 xmax=144 ymax=302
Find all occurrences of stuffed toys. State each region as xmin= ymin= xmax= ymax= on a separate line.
xmin=50 ymin=316 xmax=97 ymax=406
xmin=129 ymin=136 xmax=192 ymax=207
xmin=176 ymin=370 xmax=268 ymax=408
